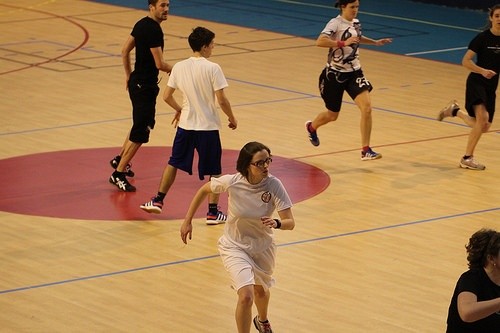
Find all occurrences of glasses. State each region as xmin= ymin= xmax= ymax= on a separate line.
xmin=250 ymin=158 xmax=273 ymax=167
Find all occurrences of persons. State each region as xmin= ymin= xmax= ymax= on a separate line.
xmin=436 ymin=4 xmax=500 ymax=170
xmin=305 ymin=0 xmax=393 ymax=162
xmin=446 ymin=229 xmax=500 ymax=333
xmin=140 ymin=27 xmax=237 ymax=225
xmin=180 ymin=142 xmax=295 ymax=333
xmin=108 ymin=0 xmax=173 ymax=192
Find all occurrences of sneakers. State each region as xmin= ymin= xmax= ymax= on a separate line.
xmin=109 ymin=156 xmax=135 ymax=177
xmin=109 ymin=172 xmax=137 ymax=192
xmin=305 ymin=121 xmax=320 ymax=146
xmin=253 ymin=315 xmax=273 ymax=333
xmin=140 ymin=198 xmax=165 ymax=214
xmin=460 ymin=156 xmax=486 ymax=170
xmin=437 ymin=99 xmax=460 ymax=122
xmin=206 ymin=210 xmax=227 ymax=224
xmin=361 ymin=148 xmax=382 ymax=161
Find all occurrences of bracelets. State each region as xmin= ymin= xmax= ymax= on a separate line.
xmin=337 ymin=41 xmax=345 ymax=48
xmin=273 ymin=219 xmax=282 ymax=229
